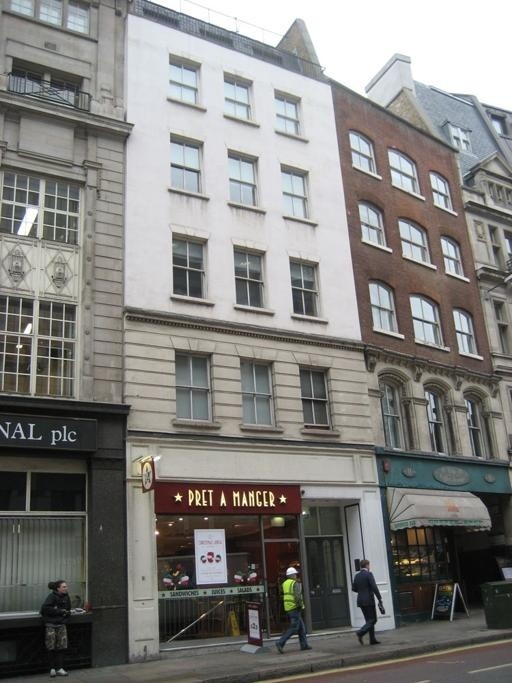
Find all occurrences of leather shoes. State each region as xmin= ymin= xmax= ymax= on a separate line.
xmin=356 ymin=632 xmax=364 ymax=645
xmin=369 ymin=640 xmax=381 ymax=644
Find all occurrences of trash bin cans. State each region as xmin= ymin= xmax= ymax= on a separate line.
xmin=481 ymin=581 xmax=511 ymax=630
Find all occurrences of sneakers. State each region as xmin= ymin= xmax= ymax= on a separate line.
xmin=275 ymin=641 xmax=284 ymax=654
xmin=300 ymin=644 xmax=312 ymax=649
xmin=50 ymin=669 xmax=56 ymax=676
xmin=56 ymin=668 xmax=68 ymax=676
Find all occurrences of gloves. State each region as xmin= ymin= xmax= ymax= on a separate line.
xmin=59 ymin=609 xmax=70 ymax=617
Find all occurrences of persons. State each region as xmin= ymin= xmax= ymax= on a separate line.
xmin=274 ymin=565 xmax=312 ymax=654
xmin=351 ymin=558 xmax=384 ymax=645
xmin=39 ymin=579 xmax=72 ymax=677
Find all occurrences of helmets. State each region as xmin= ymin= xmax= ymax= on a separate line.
xmin=286 ymin=566 xmax=298 ymax=575
